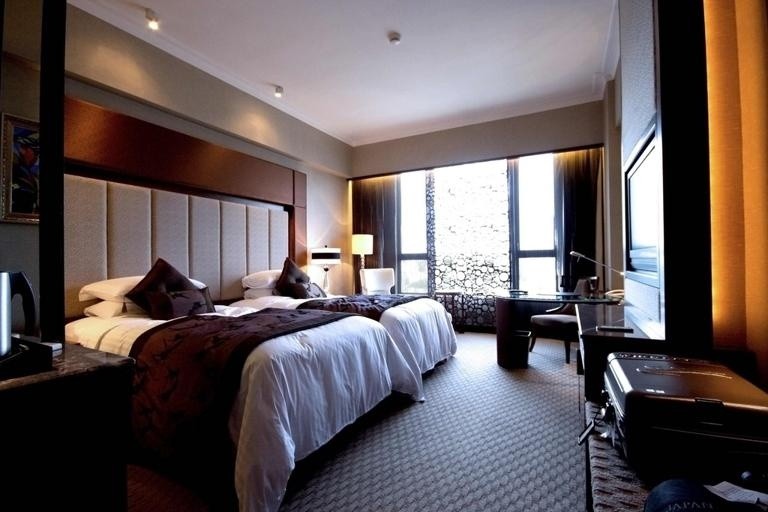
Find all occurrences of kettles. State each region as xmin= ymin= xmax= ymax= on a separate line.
xmin=0 ymin=270 xmax=40 ymax=361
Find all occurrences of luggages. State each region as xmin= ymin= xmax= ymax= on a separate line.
xmin=579 ymin=351 xmax=767 ymax=490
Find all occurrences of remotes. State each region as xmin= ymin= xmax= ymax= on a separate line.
xmin=596 ymin=325 xmax=635 ymax=332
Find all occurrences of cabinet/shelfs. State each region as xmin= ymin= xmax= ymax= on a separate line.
xmin=0 ymin=336 xmax=138 ymax=511
xmin=572 ymin=303 xmax=663 ymax=404
xmin=570 ymin=93 xmax=716 ymax=391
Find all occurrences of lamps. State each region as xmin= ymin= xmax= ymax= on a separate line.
xmin=274 ymin=85 xmax=283 ymax=98
xmin=350 ymin=233 xmax=374 ymax=269
xmin=144 ymin=6 xmax=159 ymax=31
xmin=568 ymin=251 xmax=624 ymax=276
xmin=310 ymin=245 xmax=342 ymax=298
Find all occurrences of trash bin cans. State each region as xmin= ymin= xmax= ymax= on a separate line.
xmin=516 ymin=331 xmax=531 ymax=368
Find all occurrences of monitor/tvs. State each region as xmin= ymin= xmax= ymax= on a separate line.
xmin=625 ymin=136 xmax=657 ymax=274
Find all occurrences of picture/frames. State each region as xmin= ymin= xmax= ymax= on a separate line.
xmin=1 ymin=110 xmax=44 ymax=227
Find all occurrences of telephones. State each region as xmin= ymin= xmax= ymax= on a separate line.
xmin=605 ymin=289 xmax=624 ymax=301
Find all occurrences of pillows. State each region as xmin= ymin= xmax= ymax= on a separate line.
xmin=240 ymin=255 xmax=328 ymax=303
xmin=74 ymin=258 xmax=216 ymax=321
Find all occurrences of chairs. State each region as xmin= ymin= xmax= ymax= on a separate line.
xmin=359 ymin=267 xmax=395 ymax=296
xmin=525 ymin=275 xmax=603 ymax=364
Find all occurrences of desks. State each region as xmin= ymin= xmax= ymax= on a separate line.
xmin=579 ymin=402 xmax=651 ymax=512
xmin=491 ymin=289 xmax=630 ymax=374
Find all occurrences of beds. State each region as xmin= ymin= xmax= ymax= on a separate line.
xmin=62 ymin=300 xmax=398 ymax=509
xmin=228 ymin=288 xmax=457 ymax=382
